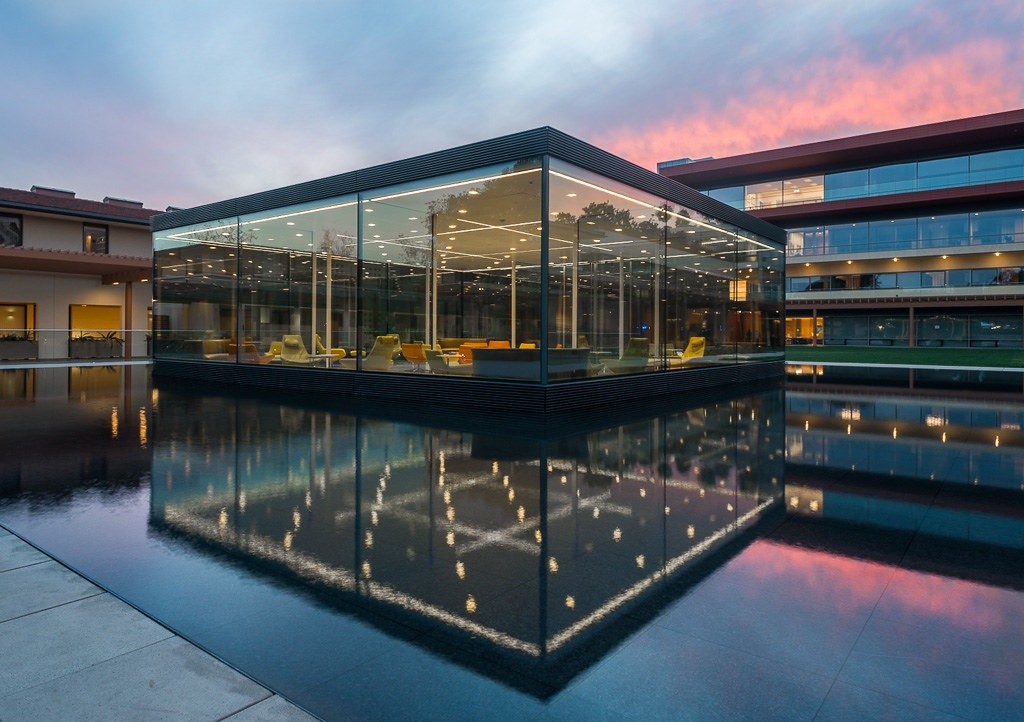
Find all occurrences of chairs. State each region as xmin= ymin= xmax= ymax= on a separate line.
xmin=599 ymin=337 xmax=649 ymax=376
xmin=225 ymin=334 xmax=319 ymax=366
xmin=340 ymin=332 xmax=535 ymax=375
xmin=314 ymin=335 xmax=346 ymax=367
xmin=669 ymin=335 xmax=705 ymax=371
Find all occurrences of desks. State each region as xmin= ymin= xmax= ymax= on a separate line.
xmin=651 ymin=355 xmax=684 ymax=372
xmin=589 ymin=351 xmax=611 ymax=367
xmin=309 ymin=354 xmax=340 ymax=368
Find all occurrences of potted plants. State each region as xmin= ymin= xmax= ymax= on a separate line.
xmin=0 ymin=326 xmax=40 ymax=363
xmin=70 ymin=330 xmax=128 ymax=361
